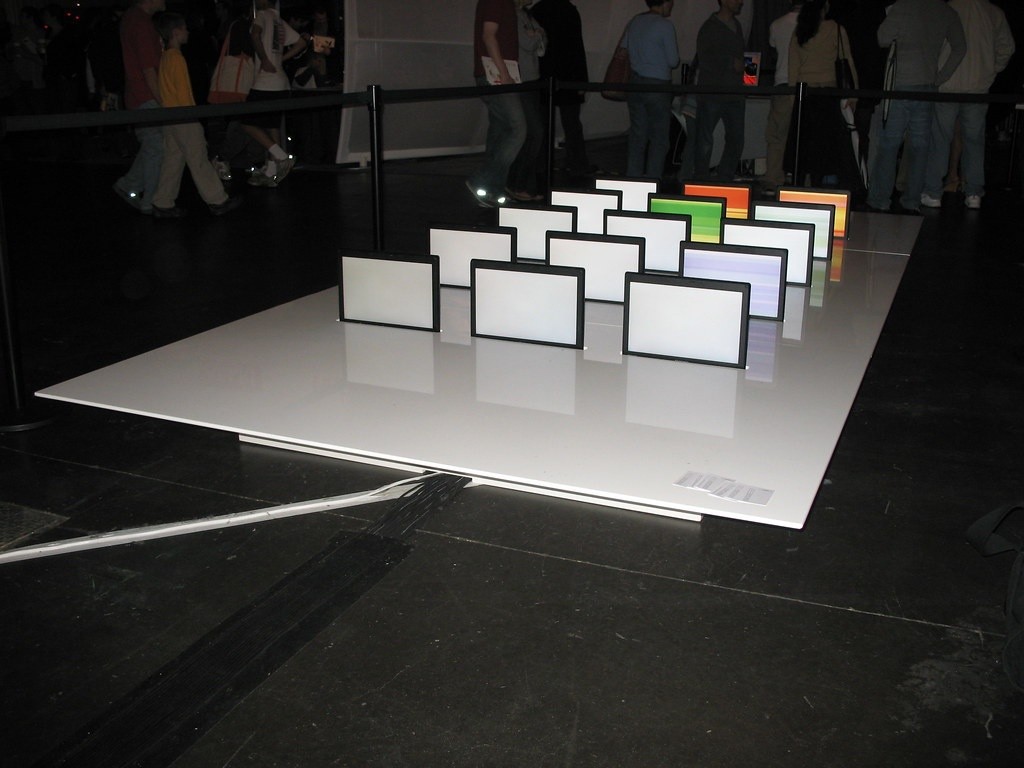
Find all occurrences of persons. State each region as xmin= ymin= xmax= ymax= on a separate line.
xmin=464 ymin=0 xmax=1015 ymax=214
xmin=0 ymin=0 xmax=342 ymax=222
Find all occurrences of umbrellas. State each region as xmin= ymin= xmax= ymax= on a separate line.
xmin=880 ymin=38 xmax=898 ymax=128
xmin=839 ymin=85 xmax=868 ymax=192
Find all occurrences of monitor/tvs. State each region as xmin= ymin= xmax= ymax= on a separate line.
xmin=337 ymin=175 xmax=852 ymax=367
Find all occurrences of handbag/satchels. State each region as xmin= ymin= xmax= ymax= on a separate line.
xmin=601 ymin=17 xmax=632 ymax=101
xmin=207 ymin=20 xmax=255 ymax=104
xmin=834 ymin=21 xmax=856 ymax=98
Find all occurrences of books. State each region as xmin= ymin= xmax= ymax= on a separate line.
xmin=481 ymin=56 xmax=523 ymax=86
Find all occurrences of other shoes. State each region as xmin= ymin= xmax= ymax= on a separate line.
xmin=153 ymin=203 xmax=186 ymax=218
xmin=274 ymin=153 xmax=296 ymax=182
xmin=920 ymin=191 xmax=941 ymax=208
xmin=247 ymin=172 xmax=279 ymax=188
xmin=210 ymin=155 xmax=232 ymax=180
xmin=210 ymin=195 xmax=243 ymax=216
xmin=465 ymin=179 xmax=504 ymax=207
xmin=505 ymin=183 xmax=534 ymax=202
xmin=550 ymin=167 xmax=585 ymax=188
xmin=964 ymin=194 xmax=981 ymax=209
xmin=114 ymin=177 xmax=153 ymax=212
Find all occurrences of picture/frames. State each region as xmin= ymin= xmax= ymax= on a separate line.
xmin=334 ymin=171 xmax=850 ymax=369
